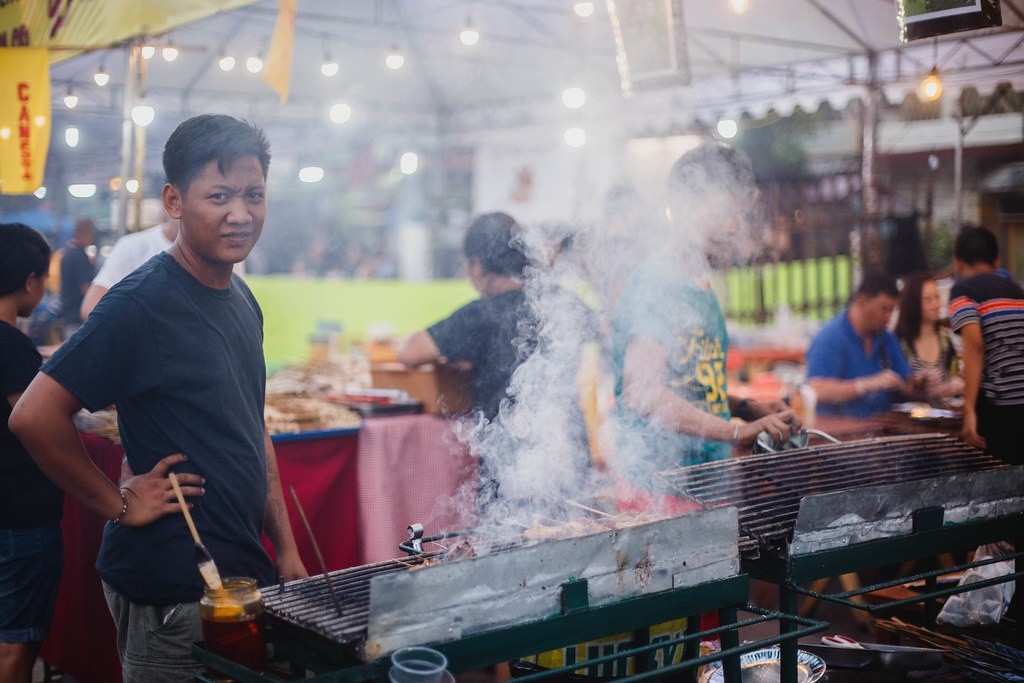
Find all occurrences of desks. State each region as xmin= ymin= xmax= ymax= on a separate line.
xmin=38 ymin=412 xmax=476 ymax=683
xmin=733 ymin=414 xmax=902 ymax=633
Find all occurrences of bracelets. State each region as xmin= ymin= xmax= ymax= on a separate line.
xmin=112 ymin=494 xmax=127 ymax=524
xmin=852 ymin=378 xmax=866 ymax=396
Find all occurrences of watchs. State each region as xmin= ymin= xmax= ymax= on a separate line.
xmin=733 ymin=425 xmax=740 ymax=446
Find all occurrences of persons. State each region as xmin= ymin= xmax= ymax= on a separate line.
xmin=395 ymin=140 xmax=1024 ymax=683
xmin=1 ymin=112 xmax=314 ymax=682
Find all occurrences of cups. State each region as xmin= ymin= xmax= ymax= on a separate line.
xmin=392 ymin=648 xmax=448 ymax=682
xmin=388 ymin=660 xmax=456 ymax=683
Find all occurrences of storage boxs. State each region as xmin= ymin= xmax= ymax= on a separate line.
xmin=371 ymin=369 xmax=471 ymax=416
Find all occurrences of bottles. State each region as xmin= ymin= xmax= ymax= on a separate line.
xmin=199 ymin=577 xmax=268 ymax=683
xmin=727 ymin=250 xmax=850 ymax=314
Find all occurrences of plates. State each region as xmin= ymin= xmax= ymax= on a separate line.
xmin=707 ymin=648 xmax=826 ymax=683
